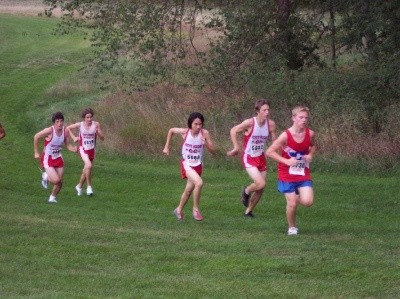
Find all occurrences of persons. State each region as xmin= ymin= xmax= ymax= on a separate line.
xmin=263 ymin=105 xmax=317 ymax=236
xmin=34 ymin=112 xmax=78 ymax=203
xmin=0 ymin=123 xmax=6 ymax=140
xmin=67 ymin=108 xmax=104 ymax=197
xmin=163 ymin=112 xmax=220 ymax=221
xmin=227 ymin=97 xmax=285 ymax=218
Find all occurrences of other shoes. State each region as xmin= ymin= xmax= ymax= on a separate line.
xmin=242 ymin=210 xmax=254 ymax=218
xmin=86 ymin=185 xmax=94 ymax=196
xmin=173 ymin=208 xmax=184 ymax=221
xmin=192 ymin=209 xmax=204 ymax=221
xmin=76 ymin=184 xmax=83 ymax=197
xmin=242 ymin=185 xmax=251 ymax=207
xmin=49 ymin=194 xmax=57 ymax=203
xmin=42 ymin=172 xmax=47 ymax=189
xmin=287 ymin=226 xmax=298 ymax=236
